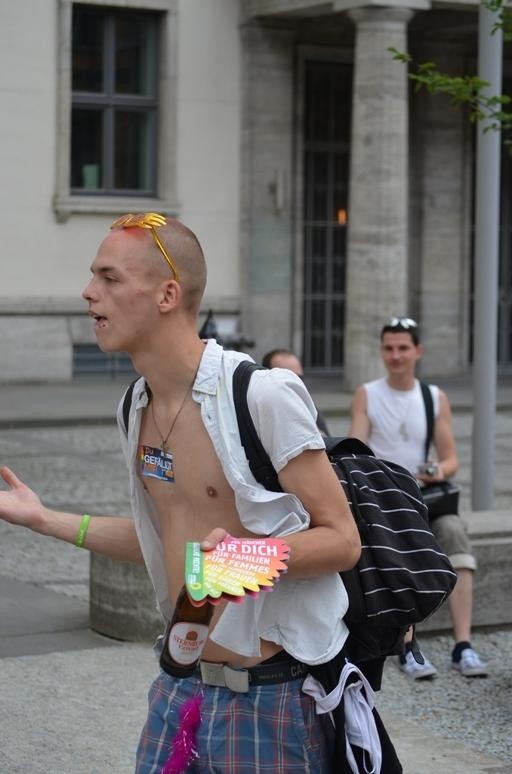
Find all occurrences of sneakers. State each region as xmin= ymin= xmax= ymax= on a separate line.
xmin=451 ymin=646 xmax=485 ymax=676
xmin=400 ymin=649 xmax=436 ymax=679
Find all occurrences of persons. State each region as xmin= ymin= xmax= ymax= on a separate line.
xmin=349 ymin=318 xmax=492 ymax=681
xmin=259 ymin=349 xmax=331 ymax=439
xmin=0 ymin=211 xmax=362 ymax=774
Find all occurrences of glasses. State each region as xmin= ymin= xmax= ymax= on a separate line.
xmin=111 ymin=212 xmax=178 ymax=281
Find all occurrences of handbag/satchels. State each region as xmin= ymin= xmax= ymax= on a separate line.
xmin=417 ymin=481 xmax=459 ymax=517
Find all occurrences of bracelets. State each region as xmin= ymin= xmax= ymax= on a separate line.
xmin=76 ymin=514 xmax=90 ymax=548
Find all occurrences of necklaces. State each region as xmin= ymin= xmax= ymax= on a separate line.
xmin=139 ymin=363 xmax=200 ymax=483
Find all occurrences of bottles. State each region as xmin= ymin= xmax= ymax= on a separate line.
xmin=159 ymin=586 xmax=217 ymax=680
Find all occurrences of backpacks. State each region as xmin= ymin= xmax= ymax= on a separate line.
xmin=122 ymin=359 xmax=457 ymax=666
